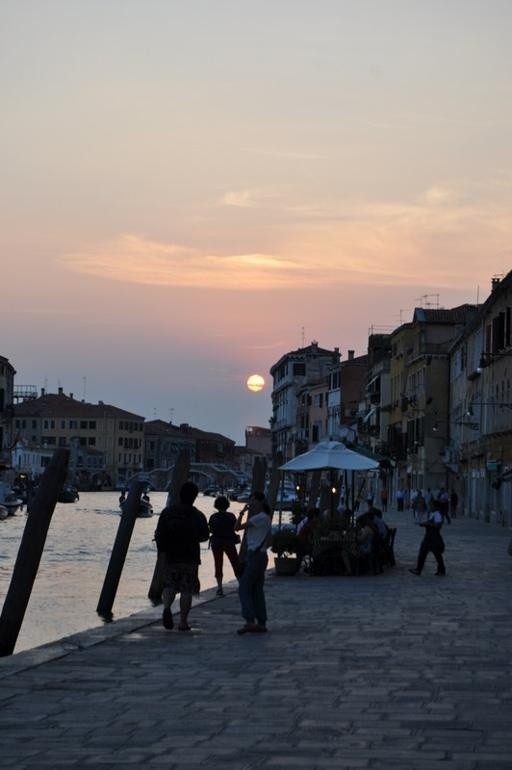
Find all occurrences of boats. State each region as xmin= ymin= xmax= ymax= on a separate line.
xmin=203 ymin=477 xmax=298 ymax=510
xmin=0 ymin=481 xmax=23 ymax=516
xmin=118 ymin=497 xmax=154 ymax=518
xmin=57 ymin=484 xmax=80 ymax=504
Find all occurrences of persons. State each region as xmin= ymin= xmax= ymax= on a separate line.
xmin=151 ymin=478 xmax=211 ymax=633
xmin=289 ymin=482 xmax=462 ymax=579
xmin=207 ymin=495 xmax=241 ymax=600
xmin=230 ymin=488 xmax=277 ymax=636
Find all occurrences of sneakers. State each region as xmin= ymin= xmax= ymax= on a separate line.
xmin=178 ymin=624 xmax=191 ymax=631
xmin=162 ymin=608 xmax=174 ymax=630
xmin=435 ymin=572 xmax=446 ymax=576
xmin=409 ymin=569 xmax=422 ymax=576
xmin=216 ymin=589 xmax=224 ymax=595
xmin=257 ymin=625 xmax=267 ymax=633
xmin=237 ymin=624 xmax=257 ymax=635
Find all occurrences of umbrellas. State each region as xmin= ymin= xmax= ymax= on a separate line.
xmin=273 ymin=438 xmax=381 ymax=520
xmin=337 ymin=438 xmax=389 ymax=511
